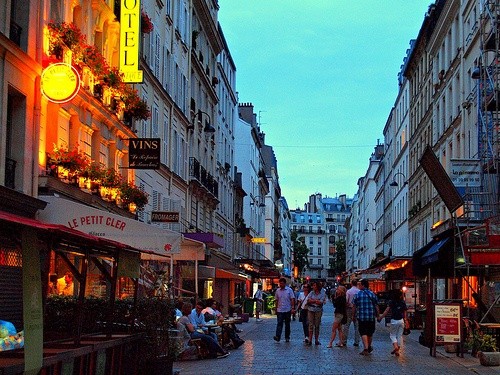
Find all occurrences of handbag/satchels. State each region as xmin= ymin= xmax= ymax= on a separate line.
xmin=299 ymin=313 xmax=302 ymax=322
xmin=403 ymin=326 xmax=410 ymax=335
xmin=341 ymin=314 xmax=348 ymax=324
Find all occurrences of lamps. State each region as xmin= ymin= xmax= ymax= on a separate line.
xmin=187 ymin=112 xmax=215 ymax=133
xmin=364 ymin=223 xmax=375 ymax=231
xmin=390 ymin=173 xmax=405 ymax=186
xmin=250 ymin=195 xmax=265 ymax=207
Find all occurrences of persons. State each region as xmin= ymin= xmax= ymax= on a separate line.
xmin=272 ymin=277 xmax=409 ymax=356
xmin=176 ymin=299 xmax=245 ymax=359
xmin=253 ymin=285 xmax=265 ymax=322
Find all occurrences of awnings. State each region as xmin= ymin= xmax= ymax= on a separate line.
xmin=216 ymin=269 xmax=246 ymax=280
xmin=363 ymin=256 xmax=412 ymax=271
xmin=37 ymin=196 xmax=205 ymax=262
xmin=421 ymin=238 xmax=451 ymax=257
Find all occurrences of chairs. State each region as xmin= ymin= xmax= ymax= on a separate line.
xmin=176 ymin=322 xmax=202 ymax=360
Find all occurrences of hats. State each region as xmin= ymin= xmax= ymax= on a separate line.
xmin=391 ymin=289 xmax=402 ymax=297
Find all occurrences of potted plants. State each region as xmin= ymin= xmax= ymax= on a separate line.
xmin=479 ymin=334 xmax=499 ymax=352
xmin=268 ymin=296 xmax=276 ymax=315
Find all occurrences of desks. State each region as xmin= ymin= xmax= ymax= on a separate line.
xmin=219 ymin=321 xmax=233 ymax=348
xmin=198 ymin=324 xmax=217 ymax=359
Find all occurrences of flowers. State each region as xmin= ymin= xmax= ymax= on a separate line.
xmin=141 ymin=10 xmax=155 ymax=34
xmin=47 ymin=16 xmax=152 ymax=123
xmin=47 ymin=143 xmax=149 ymax=208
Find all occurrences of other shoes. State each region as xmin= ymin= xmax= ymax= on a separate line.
xmin=336 ymin=343 xmax=346 ymax=346
xmin=391 ymin=346 xmax=400 ymax=356
xmin=306 ymin=343 xmax=311 ymax=346
xmin=303 ymin=336 xmax=308 ymax=342
xmin=340 ymin=344 xmax=343 ymax=347
xmin=222 ymin=344 xmax=230 ymax=348
xmin=256 ymin=318 xmax=263 ymax=321
xmin=359 ymin=346 xmax=373 ymax=356
xmin=327 ymin=345 xmax=332 ymax=347
xmin=353 ymin=343 xmax=358 ymax=347
xmin=236 ymin=329 xmax=243 ymax=333
xmin=216 ymin=349 xmax=230 ymax=359
xmin=286 ymin=339 xmax=289 ymax=342
xmin=273 ymin=336 xmax=280 ymax=341
xmin=235 ymin=340 xmax=245 ymax=349
xmin=315 ymin=341 xmax=321 ymax=345
